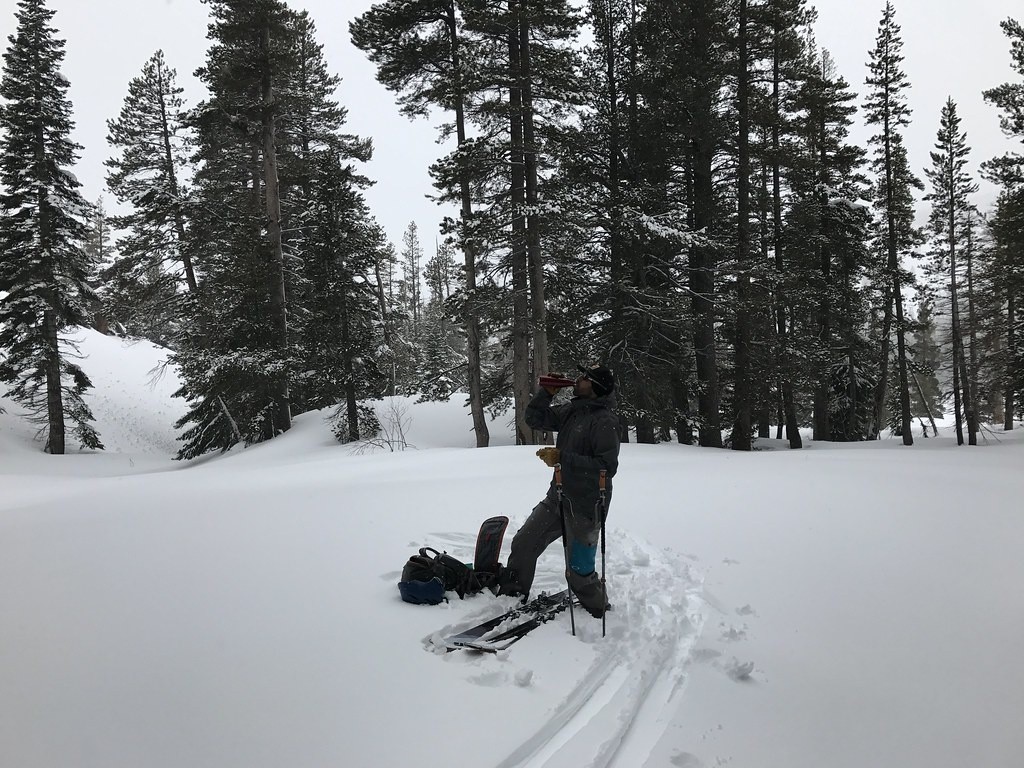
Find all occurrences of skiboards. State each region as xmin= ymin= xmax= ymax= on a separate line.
xmin=427 ymin=585 xmax=581 ymax=656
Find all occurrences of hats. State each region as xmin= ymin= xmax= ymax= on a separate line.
xmin=576 ymin=362 xmax=614 ymax=391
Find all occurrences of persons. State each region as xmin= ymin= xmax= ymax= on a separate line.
xmin=497 ymin=362 xmax=621 ymax=617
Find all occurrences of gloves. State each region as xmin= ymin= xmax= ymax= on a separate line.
xmin=536 ymin=446 xmax=561 ymax=467
xmin=544 ymin=370 xmax=565 ymax=395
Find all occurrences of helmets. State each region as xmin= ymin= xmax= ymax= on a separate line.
xmin=398 ymin=577 xmax=445 ymax=605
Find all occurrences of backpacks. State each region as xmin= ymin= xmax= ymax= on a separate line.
xmin=401 ymin=545 xmax=473 ymax=590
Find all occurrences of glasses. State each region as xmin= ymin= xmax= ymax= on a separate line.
xmin=579 ymin=371 xmax=590 ymax=380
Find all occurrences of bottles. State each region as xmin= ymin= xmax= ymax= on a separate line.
xmin=538 ymin=376 xmax=577 ymax=388
